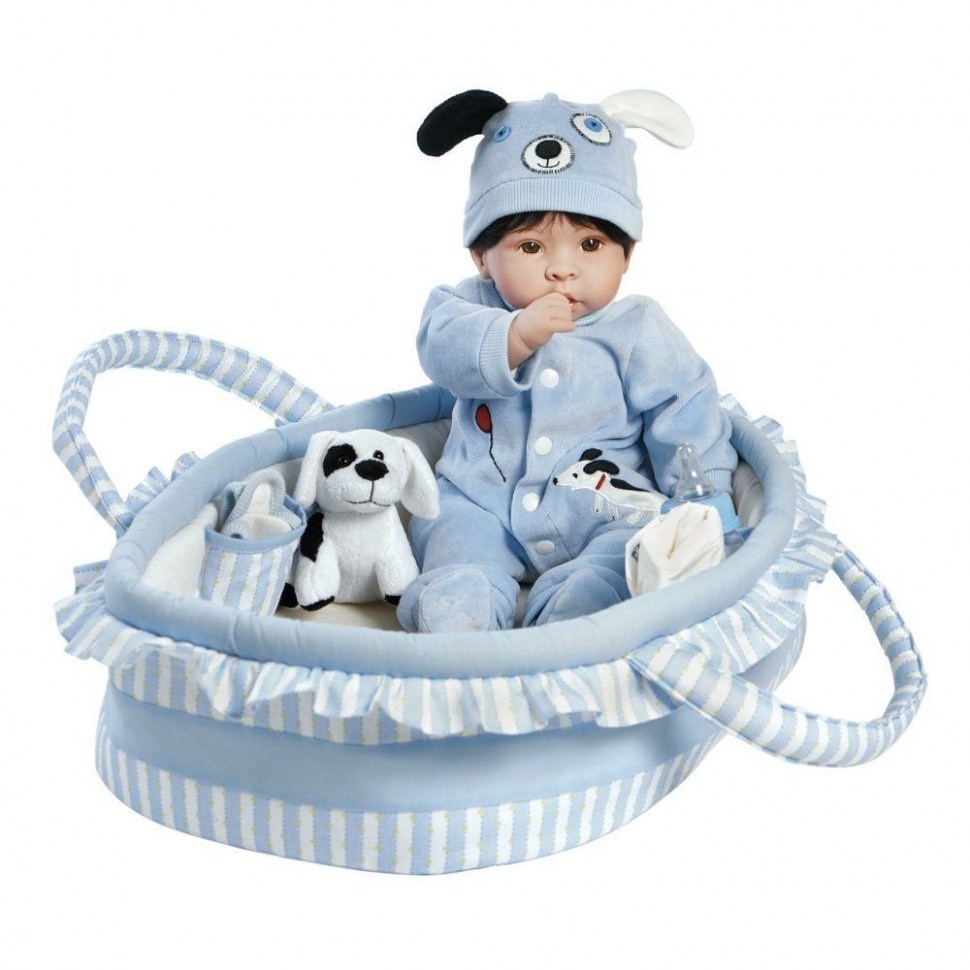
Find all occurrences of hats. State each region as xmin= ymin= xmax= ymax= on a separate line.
xmin=418 ymin=90 xmax=696 ymax=241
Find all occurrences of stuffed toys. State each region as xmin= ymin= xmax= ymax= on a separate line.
xmin=279 ymin=430 xmax=442 ymax=614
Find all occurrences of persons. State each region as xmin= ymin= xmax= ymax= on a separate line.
xmin=393 ymin=88 xmax=743 ymax=633
